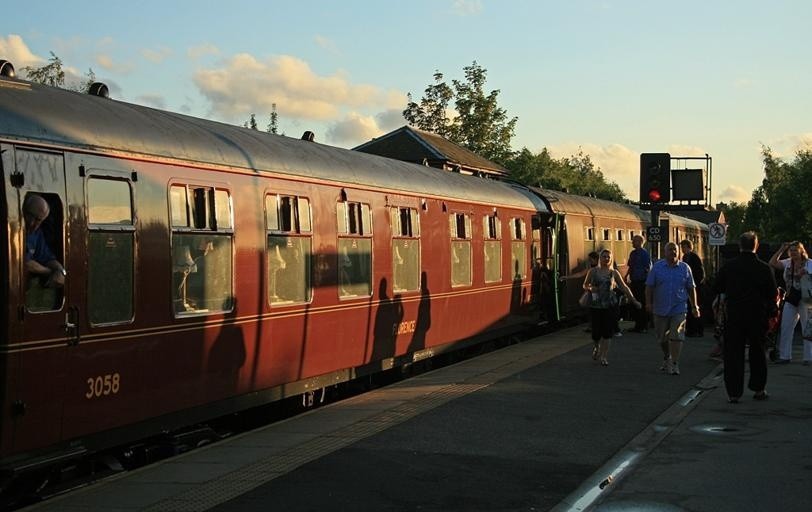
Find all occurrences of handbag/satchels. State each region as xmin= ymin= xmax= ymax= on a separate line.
xmin=578 ymin=289 xmax=592 ymax=308
xmin=784 ymin=286 xmax=802 ymax=308
xmin=613 ymin=283 xmax=632 ymax=321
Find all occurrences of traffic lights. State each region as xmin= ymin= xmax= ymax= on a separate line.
xmin=646 ymin=160 xmax=662 ymax=203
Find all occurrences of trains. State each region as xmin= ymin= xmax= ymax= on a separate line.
xmin=1 ymin=59 xmax=722 ymax=511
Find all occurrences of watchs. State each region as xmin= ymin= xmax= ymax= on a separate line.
xmin=55 ymin=268 xmax=67 ymax=276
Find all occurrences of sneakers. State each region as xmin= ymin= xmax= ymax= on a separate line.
xmin=775 ymin=359 xmax=792 ymax=364
xmin=592 ymin=344 xmax=609 ymax=367
xmin=752 ymin=390 xmax=771 ymax=401
xmin=659 ymin=352 xmax=679 ymax=375
xmin=727 ymin=396 xmax=742 ymax=404
xmin=802 ymin=360 xmax=811 ymax=366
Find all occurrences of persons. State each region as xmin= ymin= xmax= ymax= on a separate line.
xmin=24 ymin=195 xmax=66 ymax=309
xmin=559 ymin=234 xmax=811 ymax=376
xmin=711 ymin=232 xmax=777 ymax=403
